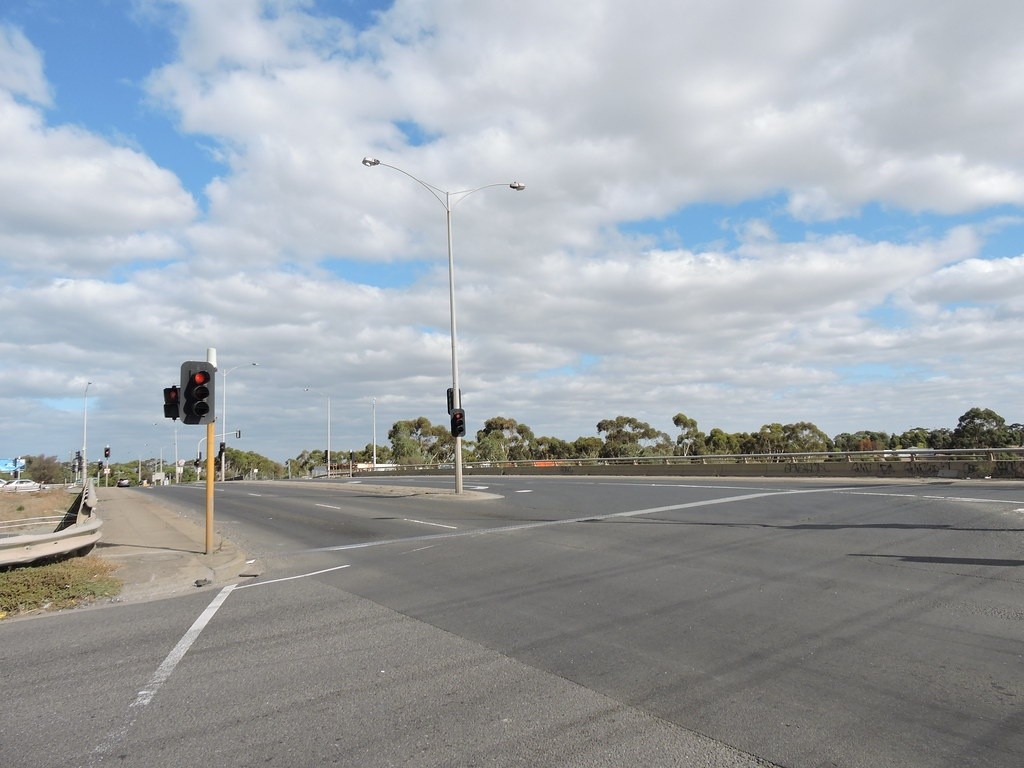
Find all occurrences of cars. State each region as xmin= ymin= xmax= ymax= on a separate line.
xmin=0 ymin=477 xmax=51 ymax=494
xmin=118 ymin=478 xmax=130 ymax=487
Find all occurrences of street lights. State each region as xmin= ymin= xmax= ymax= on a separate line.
xmin=214 ymin=362 xmax=260 ymax=482
xmin=304 ymin=382 xmax=347 ymax=478
xmin=81 ymin=381 xmax=94 ymax=488
xmin=153 ymin=422 xmax=186 ymax=485
xmin=361 ymin=156 xmax=527 ymax=499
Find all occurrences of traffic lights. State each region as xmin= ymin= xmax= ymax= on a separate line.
xmin=219 ymin=442 xmax=226 ymax=453
xmin=104 ymin=447 xmax=110 ymax=458
xmin=98 ymin=461 xmax=103 ymax=470
xmin=450 ymin=408 xmax=466 ymax=437
xmin=164 ymin=385 xmax=180 ymax=419
xmin=179 ymin=360 xmax=216 ymax=424
